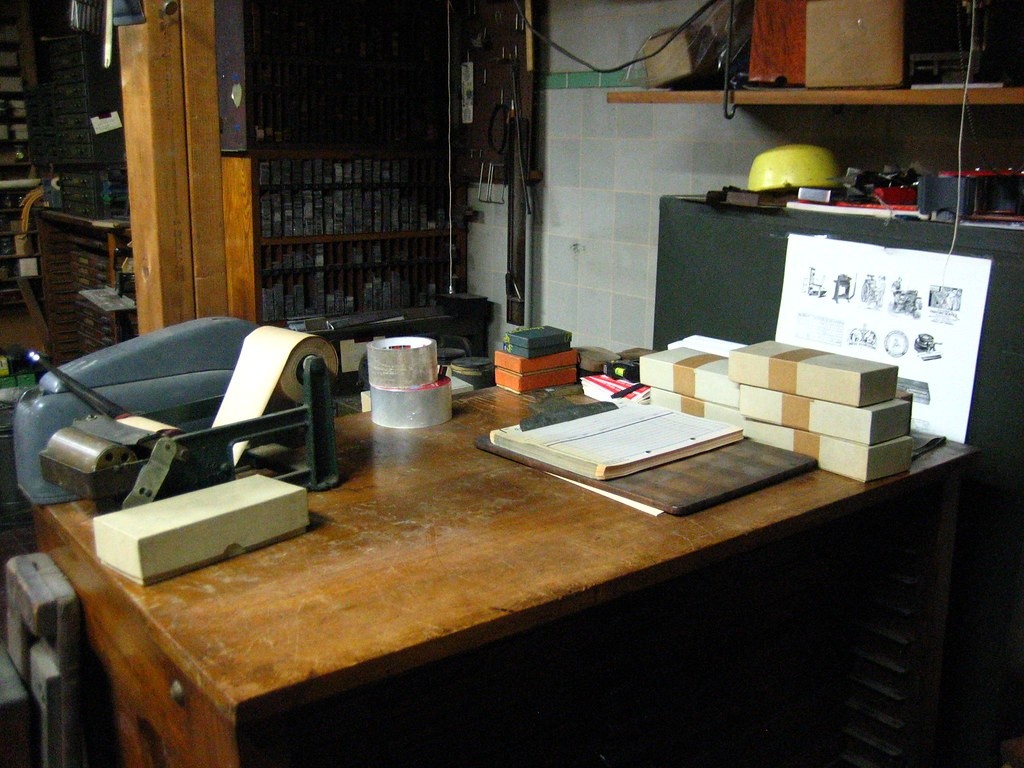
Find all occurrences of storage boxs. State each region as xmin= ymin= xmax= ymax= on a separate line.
xmin=643 ymin=26 xmax=721 ymax=88
xmin=91 ymin=474 xmax=311 ymax=587
xmin=493 ymin=325 xmax=581 ymax=394
xmin=638 ymin=334 xmax=914 ymax=484
xmin=804 ymin=0 xmax=906 ymax=88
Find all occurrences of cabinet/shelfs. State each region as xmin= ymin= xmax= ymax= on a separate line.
xmin=0 ymin=0 xmax=198 ymax=365
xmin=606 ymin=83 xmax=1024 ymax=461
xmin=181 ymin=0 xmax=473 ymax=339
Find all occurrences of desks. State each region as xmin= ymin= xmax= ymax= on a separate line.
xmin=32 ymin=386 xmax=983 ymax=768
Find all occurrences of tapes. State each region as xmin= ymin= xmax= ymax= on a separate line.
xmin=365 ymin=337 xmax=439 ymax=389
xmin=368 ymin=374 xmax=453 ymax=428
